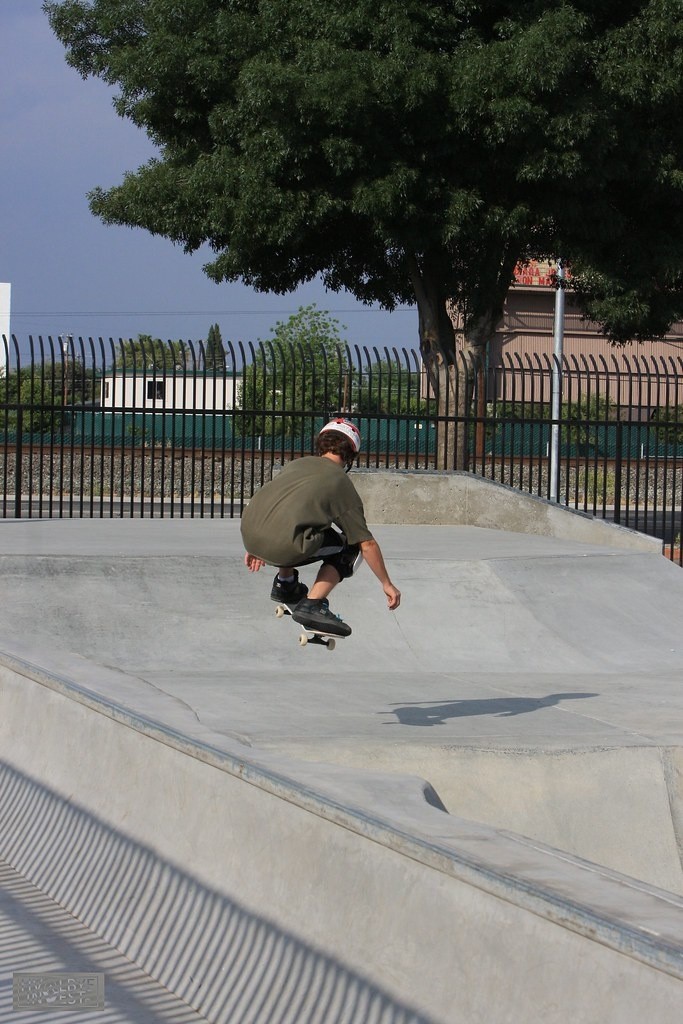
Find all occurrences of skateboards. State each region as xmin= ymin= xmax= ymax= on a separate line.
xmin=269 ymin=581 xmax=354 ymax=652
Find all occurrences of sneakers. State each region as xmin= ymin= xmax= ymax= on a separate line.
xmin=292 ymin=597 xmax=352 ymax=636
xmin=271 ymin=569 xmax=308 ymax=604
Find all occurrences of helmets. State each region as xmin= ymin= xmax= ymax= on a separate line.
xmin=318 ymin=418 xmax=361 ymax=462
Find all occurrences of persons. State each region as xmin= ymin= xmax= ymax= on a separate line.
xmin=240 ymin=418 xmax=401 ymax=638
